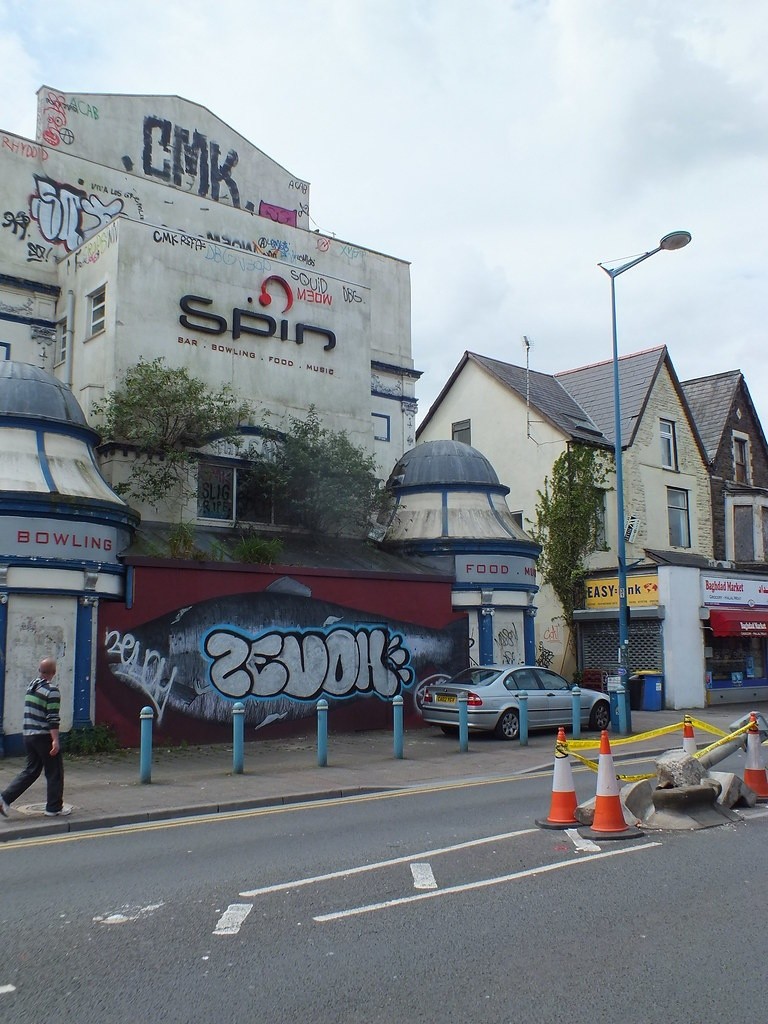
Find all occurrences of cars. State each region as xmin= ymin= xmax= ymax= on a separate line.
xmin=423 ymin=663 xmax=611 ymax=742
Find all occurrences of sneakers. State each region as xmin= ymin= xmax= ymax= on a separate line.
xmin=44 ymin=806 xmax=71 ymax=816
xmin=0 ymin=794 xmax=10 ymax=817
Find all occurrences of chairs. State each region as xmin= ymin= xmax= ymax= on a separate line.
xmin=519 ymin=675 xmax=537 ymax=689
xmin=583 ymin=668 xmax=610 ymax=696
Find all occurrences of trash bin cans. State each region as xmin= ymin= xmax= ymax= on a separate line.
xmin=629 ymin=679 xmax=642 ymax=710
xmin=633 ymin=670 xmax=664 ymax=711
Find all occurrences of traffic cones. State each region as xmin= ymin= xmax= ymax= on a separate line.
xmin=741 ymin=712 xmax=767 ymax=803
xmin=683 ymin=717 xmax=699 ymax=755
xmin=536 ymin=727 xmax=584 ymax=830
xmin=577 ymin=729 xmax=645 ymax=839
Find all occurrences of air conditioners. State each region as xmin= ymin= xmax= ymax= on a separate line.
xmin=711 ymin=560 xmax=731 ymax=569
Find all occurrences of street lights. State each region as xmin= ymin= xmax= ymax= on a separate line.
xmin=597 ymin=226 xmax=694 ymax=727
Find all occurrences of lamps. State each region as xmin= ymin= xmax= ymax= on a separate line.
xmin=80 ymin=596 xmax=98 ymax=607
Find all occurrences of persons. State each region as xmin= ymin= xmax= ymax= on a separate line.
xmin=0 ymin=658 xmax=71 ymax=818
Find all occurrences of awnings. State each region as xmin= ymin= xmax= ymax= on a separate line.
xmin=710 ymin=609 xmax=768 ymax=636
